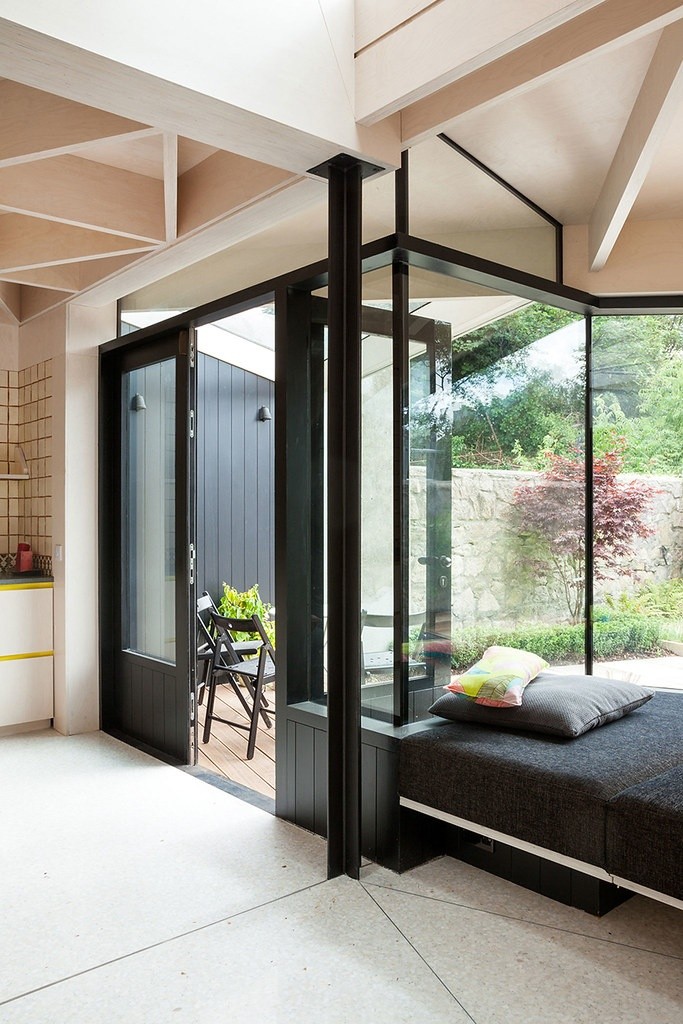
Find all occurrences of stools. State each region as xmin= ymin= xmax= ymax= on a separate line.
xmin=607 ymin=765 xmax=683 ymax=912
xmin=396 ymin=692 xmax=683 ymax=916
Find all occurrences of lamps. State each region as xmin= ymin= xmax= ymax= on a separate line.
xmin=259 ymin=405 xmax=272 ymax=423
xmin=130 ymin=393 xmax=146 ymax=411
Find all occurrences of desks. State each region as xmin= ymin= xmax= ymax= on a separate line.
xmin=0 ymin=571 xmax=54 ymax=739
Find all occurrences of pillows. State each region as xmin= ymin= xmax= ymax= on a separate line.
xmin=442 ymin=646 xmax=551 ymax=709
xmin=426 ymin=670 xmax=656 ymax=741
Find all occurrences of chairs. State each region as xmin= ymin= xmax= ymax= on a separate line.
xmin=201 ymin=606 xmax=275 ymax=760
xmin=196 ymin=591 xmax=272 ymax=729
xmin=360 ymin=609 xmax=428 ymax=687
